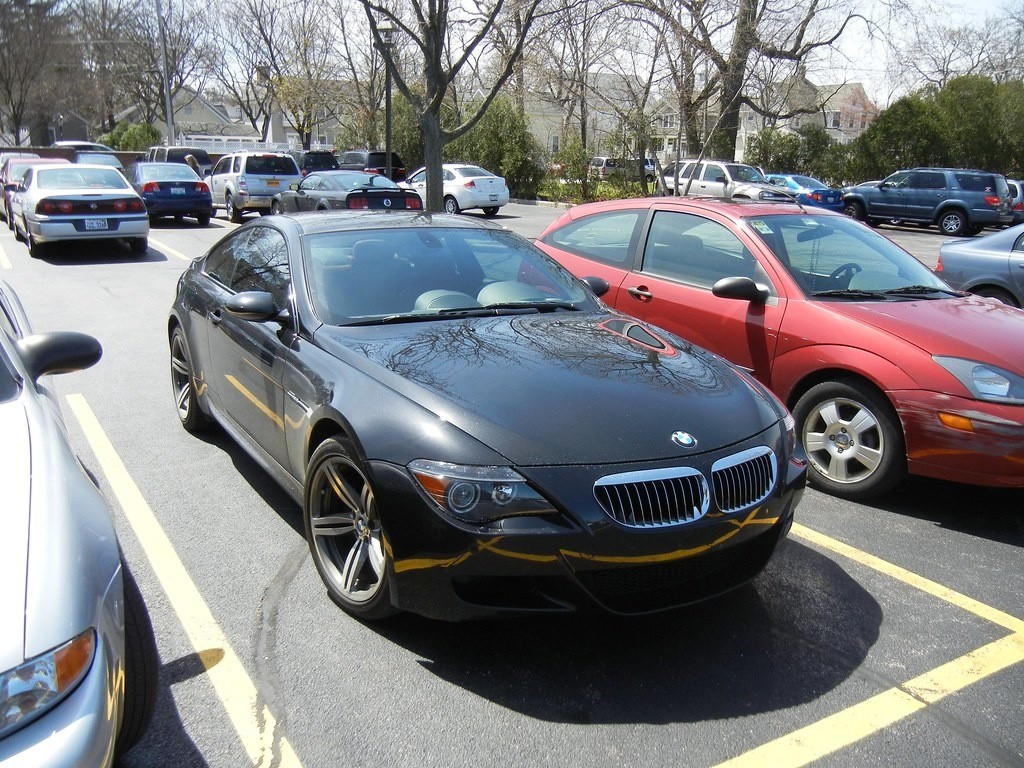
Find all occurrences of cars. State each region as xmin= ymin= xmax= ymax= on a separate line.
xmin=136 ymin=145 xmax=213 ymax=179
xmin=0 ymin=152 xmax=41 ymax=169
xmin=50 ymin=141 xmax=115 ymax=151
xmin=520 ymin=198 xmax=1024 ymax=506
xmin=931 ymin=222 xmax=1024 ymax=312
xmin=999 ymin=178 xmax=1024 ymax=224
xmin=124 ymin=162 xmax=212 ymax=225
xmin=762 ymin=173 xmax=844 ymax=214
xmin=0 ymin=157 xmax=73 ymax=217
xmin=394 ymin=163 xmax=511 ymax=216
xmin=1 ymin=273 xmax=136 ymax=768
xmin=72 ymin=151 xmax=126 ymax=174
xmin=587 ymin=156 xmax=656 ymax=182
xmin=268 ymin=170 xmax=425 ymax=211
xmin=853 ymin=179 xmax=933 ymax=227
xmin=4 ymin=164 xmax=151 ymax=258
xmin=627 ymin=158 xmax=656 ymax=171
xmin=165 ymin=208 xmax=812 ymax=622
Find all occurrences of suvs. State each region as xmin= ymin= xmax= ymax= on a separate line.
xmin=656 ymin=159 xmax=798 ymax=204
xmin=336 ymin=149 xmax=407 ymax=180
xmin=286 ymin=149 xmax=340 ymax=175
xmin=840 ymin=168 xmax=1013 ymax=236
xmin=203 ymin=150 xmax=307 ymax=222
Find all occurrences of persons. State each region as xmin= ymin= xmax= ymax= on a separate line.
xmin=410 ymin=242 xmax=478 ymax=311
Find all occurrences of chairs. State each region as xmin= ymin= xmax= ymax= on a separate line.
xmin=400 ymin=248 xmax=471 ymax=311
xmin=348 ymin=241 xmax=410 ymax=311
xmin=677 ymin=234 xmax=717 ymax=270
xmin=741 ymin=236 xmax=811 ymax=291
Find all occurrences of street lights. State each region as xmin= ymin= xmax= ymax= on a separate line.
xmin=373 ymin=15 xmax=399 ymax=181
